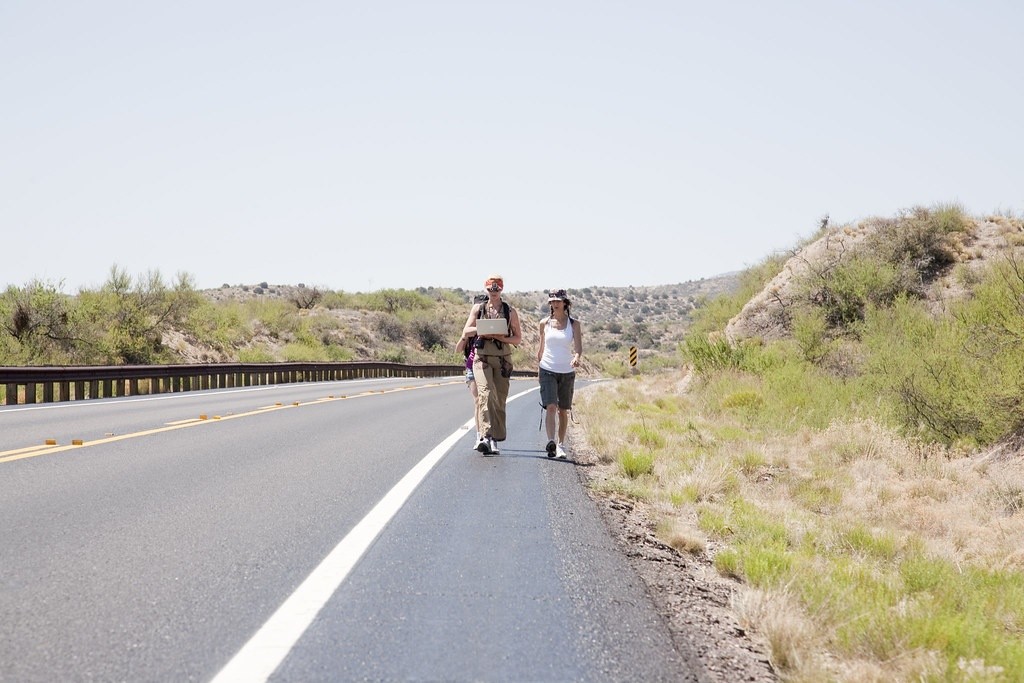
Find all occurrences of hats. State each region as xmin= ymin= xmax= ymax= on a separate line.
xmin=548 ymin=287 xmax=567 ymax=301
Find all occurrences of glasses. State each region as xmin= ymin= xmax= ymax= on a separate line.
xmin=487 ymin=287 xmax=501 ymax=292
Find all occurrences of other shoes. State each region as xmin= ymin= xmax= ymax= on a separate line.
xmin=473 ymin=438 xmax=480 ymax=450
xmin=477 ymin=437 xmax=492 ymax=455
xmin=489 ymin=441 xmax=499 ymax=455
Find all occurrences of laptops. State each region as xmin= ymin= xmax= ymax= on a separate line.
xmin=476 ymin=319 xmax=509 ymax=337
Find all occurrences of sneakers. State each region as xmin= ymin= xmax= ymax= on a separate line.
xmin=547 ymin=439 xmax=557 ymax=457
xmin=556 ymin=442 xmax=567 ymax=458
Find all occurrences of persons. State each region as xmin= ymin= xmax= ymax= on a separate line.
xmin=537 ymin=288 xmax=583 ymax=458
xmin=455 ymin=275 xmax=522 ymax=455
